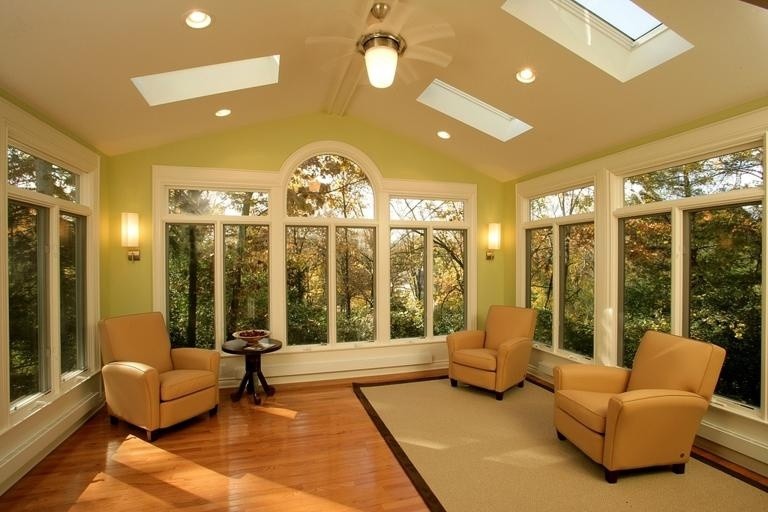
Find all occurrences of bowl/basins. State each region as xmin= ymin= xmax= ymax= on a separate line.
xmin=231 ymin=329 xmax=269 ymax=343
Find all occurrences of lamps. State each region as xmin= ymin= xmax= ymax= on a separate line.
xmin=486 ymin=222 xmax=501 ymax=262
xmin=120 ymin=211 xmax=141 ymax=263
xmin=355 ymin=2 xmax=408 ymax=89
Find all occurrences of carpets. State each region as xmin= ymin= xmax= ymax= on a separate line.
xmin=352 ymin=374 xmax=768 ymax=512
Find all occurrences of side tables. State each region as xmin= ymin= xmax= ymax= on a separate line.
xmin=221 ymin=337 xmax=283 ymax=406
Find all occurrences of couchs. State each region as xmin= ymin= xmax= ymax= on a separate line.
xmin=99 ymin=311 xmax=221 ymax=443
xmin=446 ymin=304 xmax=538 ymax=401
